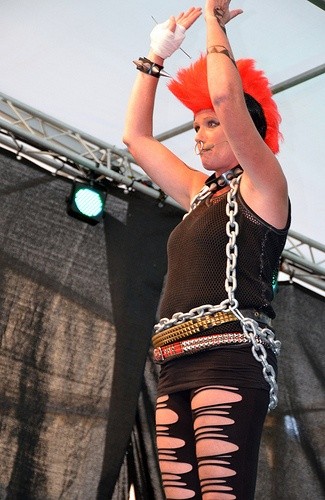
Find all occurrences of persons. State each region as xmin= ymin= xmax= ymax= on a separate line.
xmin=124 ymin=0 xmax=290 ymax=499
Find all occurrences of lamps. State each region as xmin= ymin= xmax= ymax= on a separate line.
xmin=65 ymin=179 xmax=106 ymax=225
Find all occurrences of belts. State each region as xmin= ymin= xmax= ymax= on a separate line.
xmin=154 ymin=333 xmax=271 ymax=361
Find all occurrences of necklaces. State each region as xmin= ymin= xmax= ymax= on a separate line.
xmin=204 ymin=163 xmax=243 ymax=194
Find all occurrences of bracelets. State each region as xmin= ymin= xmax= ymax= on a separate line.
xmin=133 ymin=56 xmax=170 ymax=79
